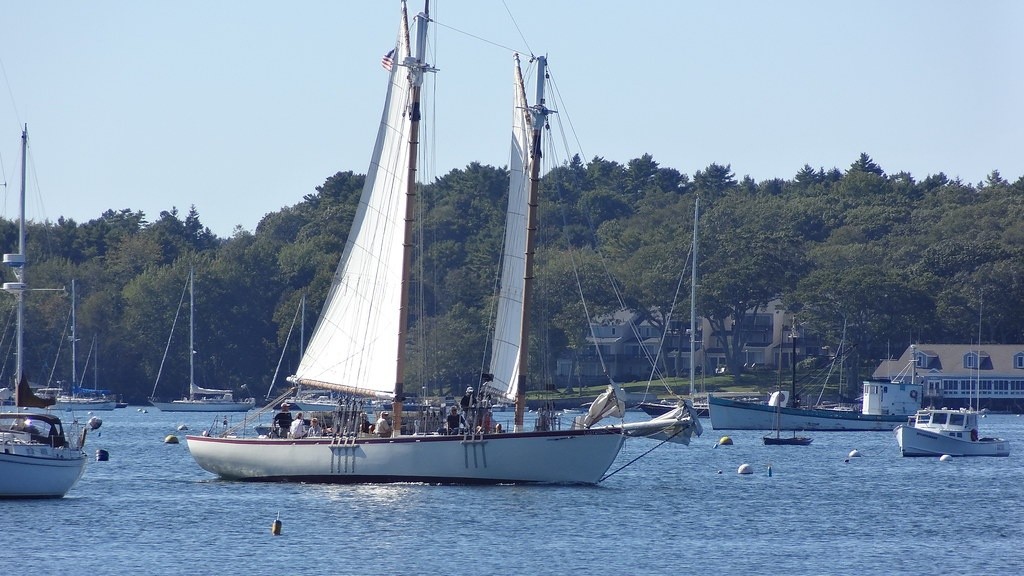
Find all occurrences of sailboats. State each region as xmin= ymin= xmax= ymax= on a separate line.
xmin=182 ymin=0 xmax=700 ymax=484
xmin=147 ymin=260 xmax=457 ymax=412
xmin=764 ymin=322 xmax=814 ymax=445
xmin=0 ymin=122 xmax=129 ymax=496
xmin=638 ymin=198 xmax=710 ymax=417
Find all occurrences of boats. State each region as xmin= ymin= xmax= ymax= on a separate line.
xmin=892 ymin=289 xmax=1011 ymax=458
xmin=707 ymin=315 xmax=923 ymax=432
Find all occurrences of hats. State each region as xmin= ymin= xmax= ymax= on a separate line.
xmin=281 ymin=403 xmax=290 ymax=407
xmin=381 ymin=411 xmax=388 ymax=415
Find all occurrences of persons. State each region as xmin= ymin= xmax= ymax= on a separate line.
xmin=461 ymin=384 xmax=476 ymax=419
xmin=289 ymin=412 xmax=308 ymax=439
xmin=273 ymin=403 xmax=294 ymax=438
xmin=446 ymin=406 xmax=467 ymax=435
xmin=308 ymin=418 xmax=322 ymax=437
xmin=360 ymin=411 xmax=391 ymax=437
